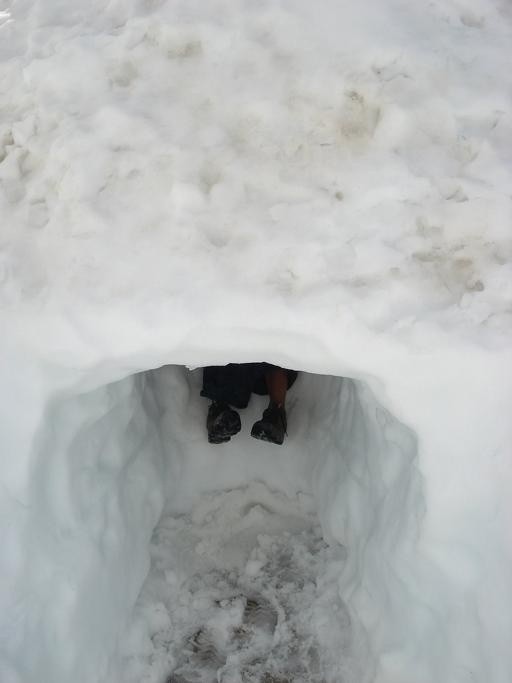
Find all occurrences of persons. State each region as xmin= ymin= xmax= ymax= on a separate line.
xmin=199 ymin=361 xmax=298 ymax=445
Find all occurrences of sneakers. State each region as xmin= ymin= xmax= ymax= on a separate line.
xmin=251 ymin=408 xmax=287 ymax=445
xmin=207 ymin=405 xmax=241 ymax=444
